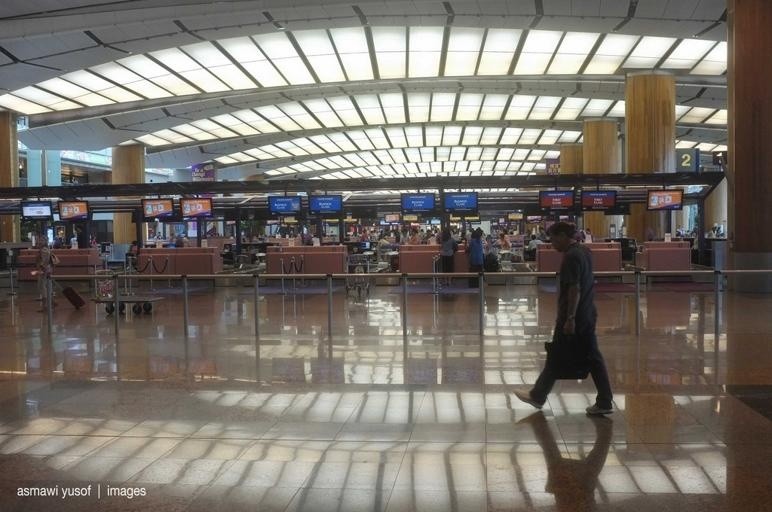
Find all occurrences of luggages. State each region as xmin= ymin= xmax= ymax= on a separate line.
xmin=53 ymin=280 xmax=85 ymax=309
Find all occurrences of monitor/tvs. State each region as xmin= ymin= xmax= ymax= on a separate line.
xmin=605 ymin=203 xmax=629 ymax=215
xmin=268 ymin=196 xmax=302 ymax=215
xmin=647 ymin=189 xmax=684 ymax=210
xmin=308 ymin=195 xmax=342 ymax=214
xmin=401 ymin=193 xmax=435 ymax=214
xmin=539 ymin=190 xmax=575 ymax=211
xmin=58 ymin=201 xmax=89 ymax=220
xmin=20 ymin=200 xmax=52 ymax=221
xmin=281 ymin=214 xmax=576 ymax=224
xmin=141 ymin=198 xmax=174 ymax=220
xmin=441 ymin=192 xmax=478 ymax=213
xmin=581 ymin=190 xmax=616 ymax=210
xmin=179 ymin=198 xmax=213 ymax=218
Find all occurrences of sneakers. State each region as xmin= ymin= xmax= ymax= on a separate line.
xmin=586 ymin=404 xmax=614 ymax=414
xmin=514 ymin=389 xmax=543 ymax=408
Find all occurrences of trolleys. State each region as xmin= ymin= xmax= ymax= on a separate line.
xmin=344 ymin=253 xmax=373 ymax=298
xmin=90 ymin=268 xmax=164 ymax=314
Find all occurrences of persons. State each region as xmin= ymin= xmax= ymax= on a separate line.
xmin=511 ymin=221 xmax=617 ymax=420
xmin=66 ymin=215 xmax=728 ymax=290
xmin=29 ymin=232 xmax=60 ymax=314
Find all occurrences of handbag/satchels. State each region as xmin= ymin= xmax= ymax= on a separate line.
xmin=545 ymin=342 xmax=588 ymax=379
xmin=50 ymin=252 xmax=60 ymax=266
